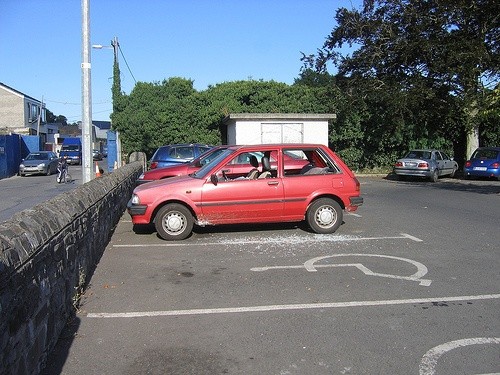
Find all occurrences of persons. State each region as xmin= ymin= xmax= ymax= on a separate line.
xmin=56 ymin=153 xmax=68 ymax=183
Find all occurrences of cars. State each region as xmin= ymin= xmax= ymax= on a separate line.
xmin=136 ymin=144 xmax=313 ymax=186
xmin=93 ymin=149 xmax=103 ymax=161
xmin=463 ymin=147 xmax=500 ymax=180
xmin=147 ymin=142 xmax=226 ymax=170
xmin=394 ymin=149 xmax=459 ymax=182
xmin=19 ymin=151 xmax=62 ymax=176
xmin=126 ymin=142 xmax=364 ymax=241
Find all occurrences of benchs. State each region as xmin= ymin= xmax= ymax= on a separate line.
xmin=299 ymin=163 xmax=331 ymax=175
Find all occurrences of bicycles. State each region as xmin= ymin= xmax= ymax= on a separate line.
xmin=56 ymin=164 xmax=72 ymax=184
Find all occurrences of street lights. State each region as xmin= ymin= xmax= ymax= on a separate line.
xmin=92 ymin=44 xmax=122 ymax=169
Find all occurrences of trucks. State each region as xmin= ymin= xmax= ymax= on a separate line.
xmin=57 ymin=137 xmax=82 ymax=165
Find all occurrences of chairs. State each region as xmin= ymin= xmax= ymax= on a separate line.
xmin=257 ymin=157 xmax=271 ymax=179
xmin=246 ymin=156 xmax=259 ymax=180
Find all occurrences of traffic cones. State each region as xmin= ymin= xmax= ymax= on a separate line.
xmin=96 ymin=162 xmax=101 ymax=177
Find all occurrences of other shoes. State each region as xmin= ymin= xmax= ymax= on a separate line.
xmin=57 ymin=178 xmax=60 ymax=182
